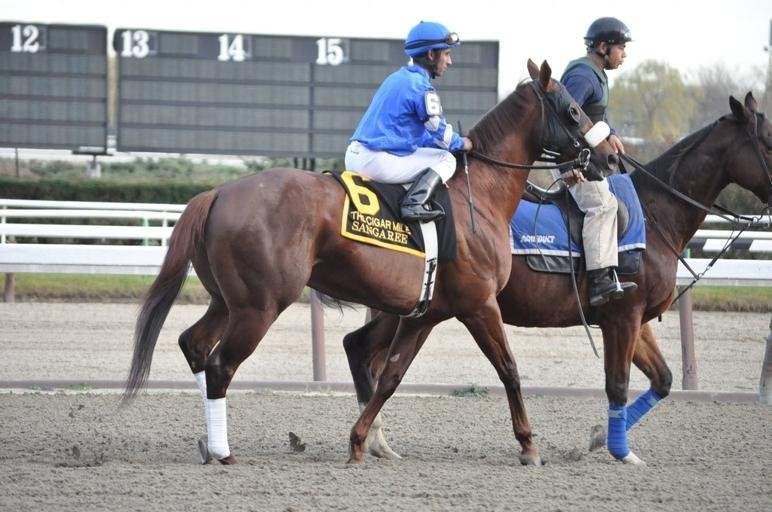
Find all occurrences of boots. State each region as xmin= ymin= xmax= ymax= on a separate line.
xmin=399 ymin=169 xmax=443 ymax=220
xmin=589 ymin=268 xmax=636 ymax=305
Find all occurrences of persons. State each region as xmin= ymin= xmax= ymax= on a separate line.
xmin=343 ymin=22 xmax=472 ymax=220
xmin=561 ymin=17 xmax=639 ymax=307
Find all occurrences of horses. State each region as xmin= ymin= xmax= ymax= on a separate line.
xmin=110 ymin=56 xmax=623 ymax=468
xmin=315 ymin=89 xmax=772 ymax=469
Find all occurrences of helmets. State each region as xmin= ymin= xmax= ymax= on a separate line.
xmin=404 ymin=22 xmax=460 ymax=55
xmin=585 ymin=17 xmax=632 ymax=47
